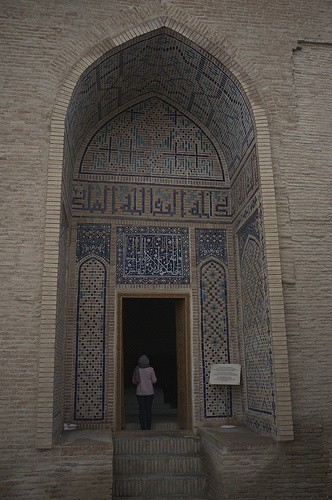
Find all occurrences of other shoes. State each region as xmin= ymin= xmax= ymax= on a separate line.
xmin=141 ymin=426 xmax=151 ymax=430
